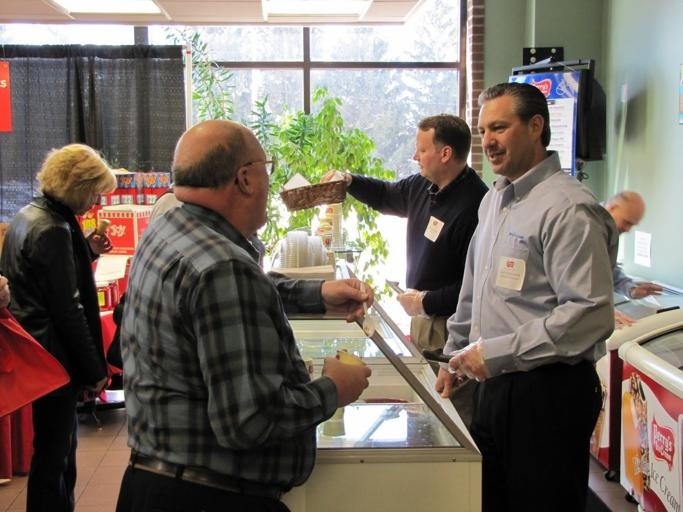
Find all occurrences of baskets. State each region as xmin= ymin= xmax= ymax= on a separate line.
xmin=280 ymin=178 xmax=348 ymax=212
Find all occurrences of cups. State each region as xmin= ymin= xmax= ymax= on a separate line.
xmin=334 ymin=349 xmax=367 ymax=370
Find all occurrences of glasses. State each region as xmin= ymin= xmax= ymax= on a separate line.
xmin=232 ymin=155 xmax=280 ymax=186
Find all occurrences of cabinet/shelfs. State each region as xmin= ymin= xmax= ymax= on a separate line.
xmin=263 ymin=258 xmax=483 ymax=512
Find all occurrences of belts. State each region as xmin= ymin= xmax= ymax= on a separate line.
xmin=128 ymin=446 xmax=286 ymax=502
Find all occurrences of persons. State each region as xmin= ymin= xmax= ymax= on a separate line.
xmin=318 ymin=112 xmax=491 ymax=448
xmin=146 ymin=187 xmax=267 ymax=283
xmin=594 ymin=189 xmax=664 ymax=302
xmin=432 ymin=81 xmax=618 ymax=512
xmin=0 ymin=274 xmax=11 ymax=310
xmin=0 ymin=143 xmax=114 ymax=512
xmin=113 ymin=116 xmax=376 ymax=512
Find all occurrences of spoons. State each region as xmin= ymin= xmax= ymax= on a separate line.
xmin=358 ymin=281 xmax=377 ymax=338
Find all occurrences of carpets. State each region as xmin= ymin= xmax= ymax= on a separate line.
xmin=586 ymin=486 xmax=613 ymax=512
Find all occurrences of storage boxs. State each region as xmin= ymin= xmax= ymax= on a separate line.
xmin=97 ymin=204 xmax=154 ymax=255
